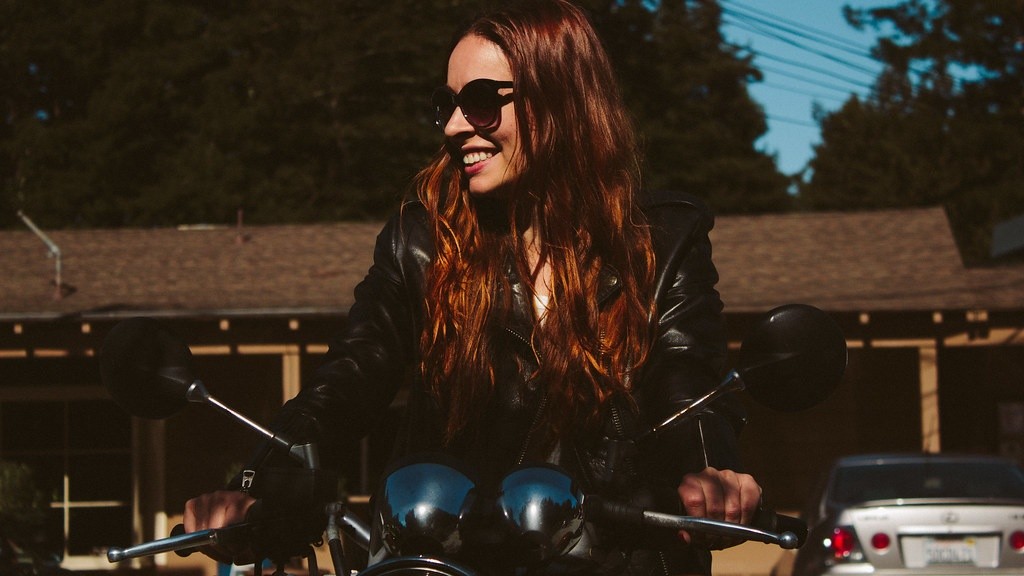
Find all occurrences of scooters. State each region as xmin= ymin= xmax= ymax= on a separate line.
xmin=96 ymin=305 xmax=855 ymax=576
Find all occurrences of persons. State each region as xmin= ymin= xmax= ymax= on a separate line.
xmin=183 ymin=0 xmax=768 ymax=576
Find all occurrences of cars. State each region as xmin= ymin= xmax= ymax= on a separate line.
xmin=790 ymin=448 xmax=1024 ymax=575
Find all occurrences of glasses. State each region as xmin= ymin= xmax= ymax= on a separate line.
xmin=430 ymin=79 xmax=515 ymax=125
